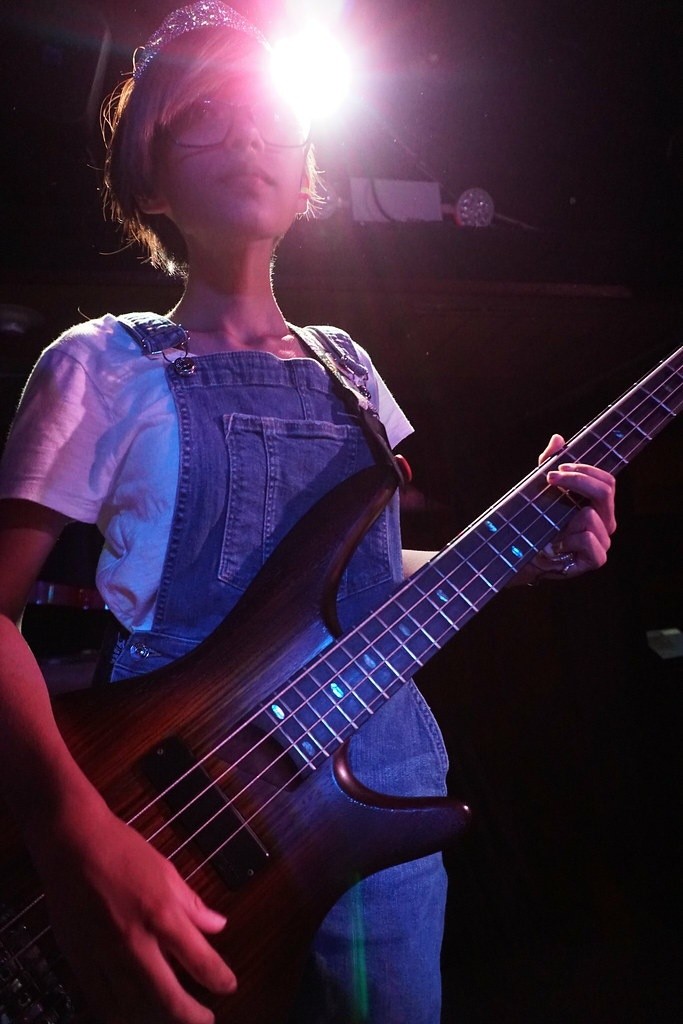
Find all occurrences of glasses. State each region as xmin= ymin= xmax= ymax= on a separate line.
xmin=153 ymin=94 xmax=314 ymax=149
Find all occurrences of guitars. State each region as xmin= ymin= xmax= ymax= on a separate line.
xmin=0 ymin=346 xmax=683 ymax=1023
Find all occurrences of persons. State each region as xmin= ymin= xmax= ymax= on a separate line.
xmin=0 ymin=3 xmax=615 ymax=1022
